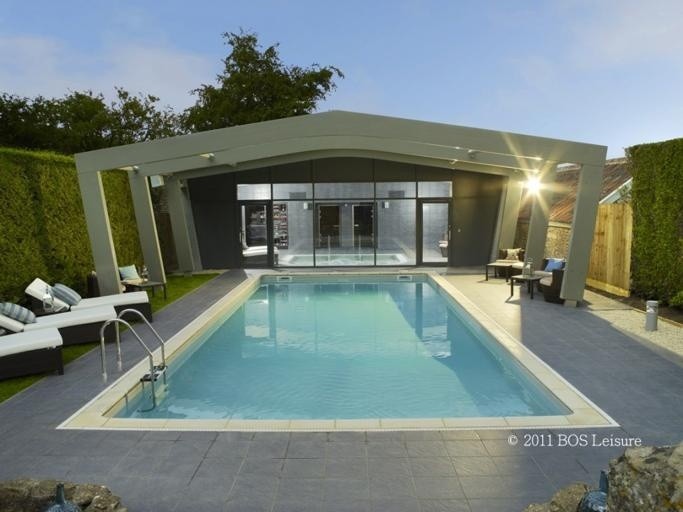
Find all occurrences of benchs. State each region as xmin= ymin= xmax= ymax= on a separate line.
xmin=242 ymin=244 xmax=278 ymax=267
xmin=0 ymin=328 xmax=64 ymax=380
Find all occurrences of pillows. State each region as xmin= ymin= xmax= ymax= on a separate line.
xmin=543 ymin=257 xmax=564 ymax=273
xmin=504 ymin=248 xmax=520 ymax=260
xmin=0 ymin=302 xmax=36 ymax=324
xmin=50 ymin=282 xmax=81 ymax=306
xmin=117 ymin=264 xmax=139 ymax=281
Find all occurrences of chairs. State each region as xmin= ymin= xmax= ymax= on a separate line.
xmin=23 ymin=277 xmax=152 ymax=322
xmin=86 ymin=272 xmax=99 ymax=298
xmin=0 ymin=302 xmax=118 ymax=345
xmin=485 ymin=249 xmax=524 ymax=282
xmin=539 ymin=269 xmax=565 ymax=304
xmin=117 ymin=267 xmax=138 ymax=292
xmin=531 ymin=257 xmax=565 ymax=293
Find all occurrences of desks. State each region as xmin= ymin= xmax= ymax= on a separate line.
xmin=509 ymin=276 xmax=541 ymax=300
xmin=126 ymin=282 xmax=166 ymax=299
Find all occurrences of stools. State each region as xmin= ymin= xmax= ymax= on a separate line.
xmin=438 ymin=239 xmax=447 ymax=257
